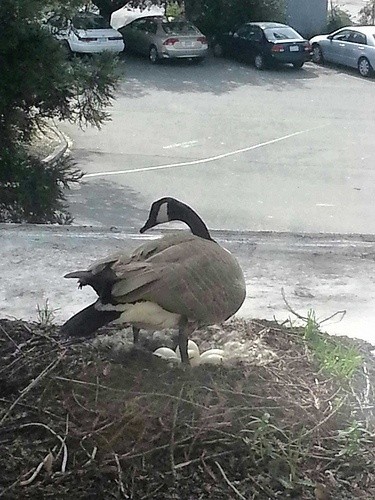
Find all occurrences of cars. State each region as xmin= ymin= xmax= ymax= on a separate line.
xmin=117 ymin=14 xmax=209 ymax=65
xmin=212 ymin=21 xmax=312 ymax=71
xmin=308 ymin=25 xmax=375 ymax=78
xmin=40 ymin=10 xmax=126 ymax=60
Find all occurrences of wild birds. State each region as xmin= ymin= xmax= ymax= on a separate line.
xmin=57 ymin=196 xmax=246 ymax=367
